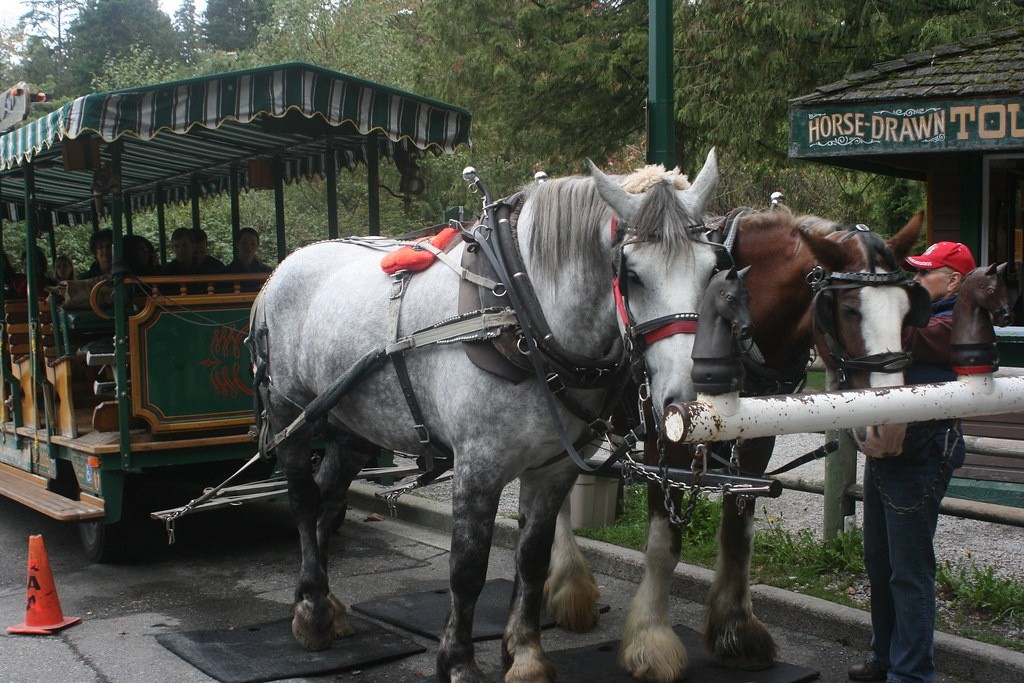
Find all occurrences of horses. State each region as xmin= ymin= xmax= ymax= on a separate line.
xmin=246 ymin=147 xmax=933 ymax=683
xmin=947 ymin=261 xmax=1011 ymax=374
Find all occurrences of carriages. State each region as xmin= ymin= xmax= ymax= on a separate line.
xmin=0 ymin=62 xmax=928 ymax=683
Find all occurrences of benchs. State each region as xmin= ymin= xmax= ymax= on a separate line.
xmin=5 ymin=301 xmax=118 ymax=439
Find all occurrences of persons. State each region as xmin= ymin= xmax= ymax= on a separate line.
xmin=52 ymin=257 xmax=75 ymax=283
xmin=164 ymin=226 xmax=226 ymax=295
xmin=7 ymin=247 xmax=52 ymax=298
xmin=78 ymin=229 xmax=161 ymax=288
xmin=848 ymin=240 xmax=978 ymax=683
xmin=221 ymin=228 xmax=274 ymax=293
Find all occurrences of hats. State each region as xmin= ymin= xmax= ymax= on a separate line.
xmin=904 ymin=241 xmax=975 ymax=275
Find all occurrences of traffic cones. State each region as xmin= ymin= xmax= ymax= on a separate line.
xmin=6 ymin=534 xmax=81 ymax=634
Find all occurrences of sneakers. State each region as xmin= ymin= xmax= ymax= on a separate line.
xmin=848 ymin=659 xmax=887 ymax=681
